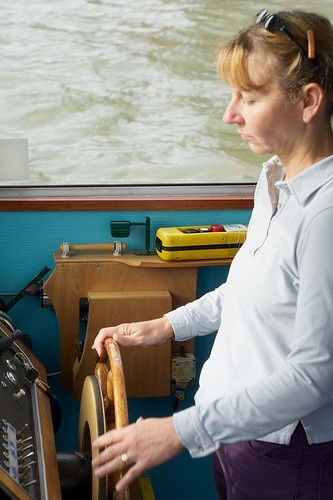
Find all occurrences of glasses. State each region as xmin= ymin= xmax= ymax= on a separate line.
xmin=253 ymin=8 xmax=305 ymax=54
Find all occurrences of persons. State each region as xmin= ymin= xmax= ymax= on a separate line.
xmin=89 ymin=8 xmax=333 ymax=500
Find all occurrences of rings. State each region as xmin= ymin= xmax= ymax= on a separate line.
xmin=121 ymin=452 xmax=132 ymax=466
